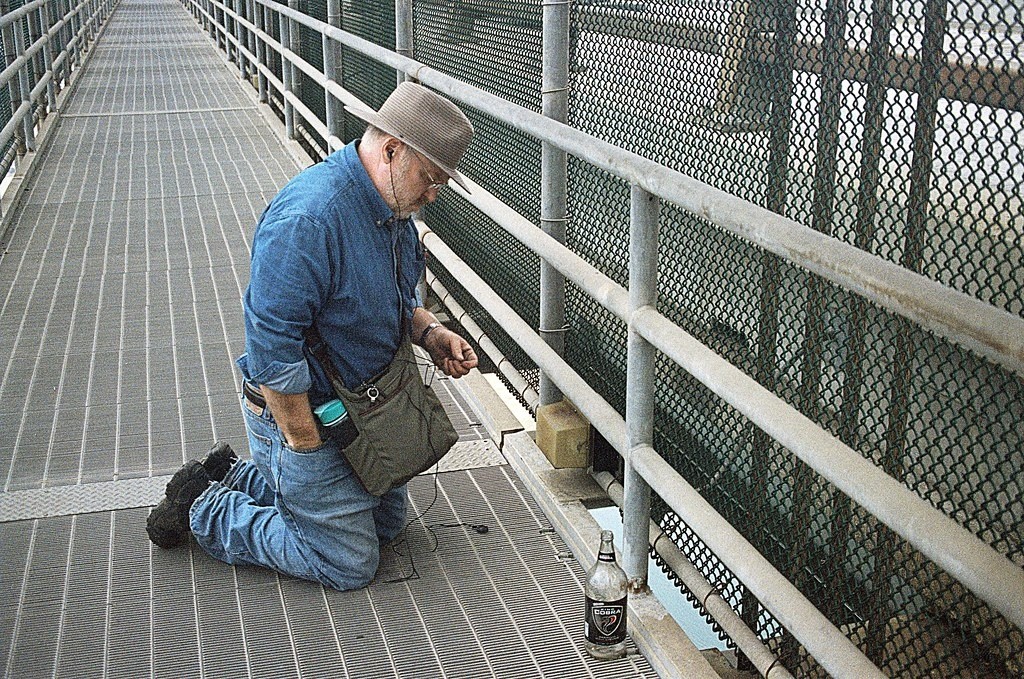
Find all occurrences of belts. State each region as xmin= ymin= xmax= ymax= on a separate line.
xmin=243 ymin=382 xmax=319 ymax=419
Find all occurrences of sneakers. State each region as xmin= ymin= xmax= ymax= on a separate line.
xmin=201 ymin=441 xmax=238 ymax=483
xmin=146 ymin=459 xmax=213 ymax=550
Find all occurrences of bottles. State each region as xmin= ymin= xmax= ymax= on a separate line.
xmin=582 ymin=529 xmax=629 ymax=662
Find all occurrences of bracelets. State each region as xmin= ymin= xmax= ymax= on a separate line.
xmin=420 ymin=322 xmax=441 ymax=350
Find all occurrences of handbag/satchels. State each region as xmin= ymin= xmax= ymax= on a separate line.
xmin=307 ymin=333 xmax=459 ymax=497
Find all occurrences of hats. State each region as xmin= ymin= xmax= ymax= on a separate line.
xmin=344 ymin=82 xmax=475 ymax=196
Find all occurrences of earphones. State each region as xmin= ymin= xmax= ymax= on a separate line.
xmin=473 ymin=525 xmax=489 ymax=533
xmin=387 ymin=148 xmax=393 ymax=158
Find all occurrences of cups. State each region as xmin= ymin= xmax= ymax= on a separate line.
xmin=313 ymin=397 xmax=360 ymax=452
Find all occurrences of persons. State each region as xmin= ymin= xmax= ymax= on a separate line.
xmin=147 ymin=82 xmax=479 ymax=592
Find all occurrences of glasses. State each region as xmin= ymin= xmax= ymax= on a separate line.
xmin=411 ymin=147 xmax=449 ymax=191
